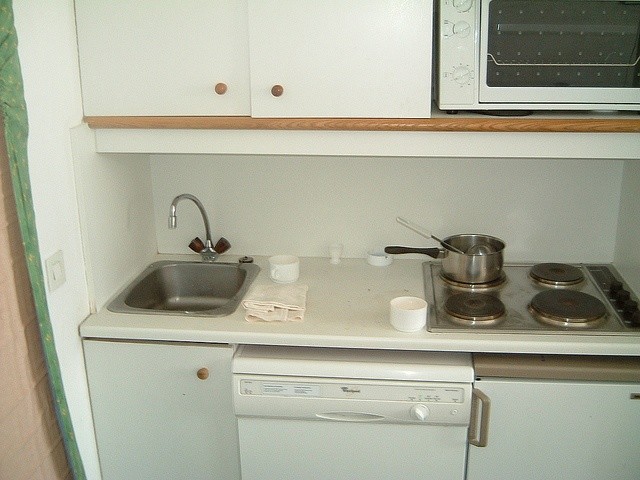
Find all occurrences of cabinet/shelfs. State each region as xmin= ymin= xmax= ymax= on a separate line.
xmin=466 ymin=377 xmax=640 ymax=480
xmin=80 ymin=338 xmax=240 ymax=480
xmin=74 ymin=1 xmax=432 ymax=129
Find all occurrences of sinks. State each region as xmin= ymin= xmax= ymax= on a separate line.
xmin=107 ymin=261 xmax=268 ymax=319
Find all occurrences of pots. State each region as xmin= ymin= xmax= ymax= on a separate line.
xmin=384 ymin=233 xmax=506 ymax=284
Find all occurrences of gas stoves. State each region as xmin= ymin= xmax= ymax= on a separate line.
xmin=422 ymin=261 xmax=640 ymax=335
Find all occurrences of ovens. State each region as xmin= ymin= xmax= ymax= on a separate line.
xmin=236 ymin=416 xmax=469 ymax=480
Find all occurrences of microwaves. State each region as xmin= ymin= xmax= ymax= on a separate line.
xmin=434 ymin=0 xmax=640 ymax=114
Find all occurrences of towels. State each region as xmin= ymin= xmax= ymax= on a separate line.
xmin=241 ymin=283 xmax=308 ymax=314
xmin=246 ymin=310 xmax=303 ymax=323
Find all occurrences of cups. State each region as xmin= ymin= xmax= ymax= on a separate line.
xmin=328 ymin=242 xmax=343 ymax=265
xmin=269 ymin=254 xmax=301 ymax=283
xmin=390 ymin=295 xmax=428 ymax=332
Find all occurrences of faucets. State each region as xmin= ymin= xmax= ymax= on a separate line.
xmin=168 ymin=196 xmax=213 ymax=258
